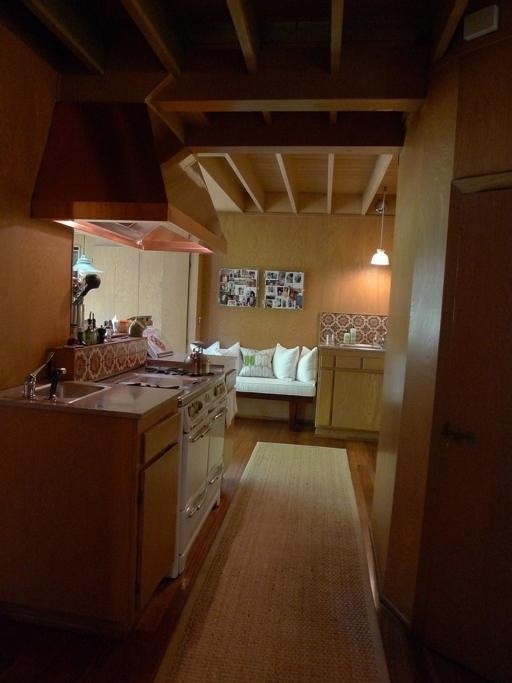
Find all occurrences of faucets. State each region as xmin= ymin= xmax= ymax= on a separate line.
xmin=374 ymin=333 xmax=386 ymax=343
xmin=49 ymin=368 xmax=67 ymax=398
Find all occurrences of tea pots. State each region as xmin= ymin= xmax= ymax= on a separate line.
xmin=184 ymin=342 xmax=212 ymax=375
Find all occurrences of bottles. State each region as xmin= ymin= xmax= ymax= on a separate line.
xmin=343 ymin=325 xmax=357 ymax=345
xmin=70 ymin=312 xmax=129 ymax=347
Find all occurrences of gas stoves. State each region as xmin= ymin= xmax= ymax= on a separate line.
xmin=96 ymin=366 xmax=228 ymax=434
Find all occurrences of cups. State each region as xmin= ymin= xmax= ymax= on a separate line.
xmin=325 ymin=334 xmax=335 ymax=346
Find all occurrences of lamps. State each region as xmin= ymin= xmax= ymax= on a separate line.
xmin=370 ymin=185 xmax=390 ymax=266
xmin=72 ymin=235 xmax=103 ymax=273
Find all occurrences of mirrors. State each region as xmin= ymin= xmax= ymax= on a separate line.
xmin=73 ymin=228 xmax=140 ymax=334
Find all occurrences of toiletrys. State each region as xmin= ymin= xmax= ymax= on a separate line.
xmin=343 ymin=329 xmax=351 ymax=344
xmin=350 ymin=325 xmax=357 ymax=343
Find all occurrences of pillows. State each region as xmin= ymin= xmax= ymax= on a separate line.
xmin=190 ymin=341 xmax=319 ymax=386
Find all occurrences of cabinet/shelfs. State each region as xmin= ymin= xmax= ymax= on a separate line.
xmin=312 ymin=345 xmax=384 ymax=441
xmin=1 ymin=397 xmax=182 ymax=641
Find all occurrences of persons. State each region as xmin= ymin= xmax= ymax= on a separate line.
xmin=246 ymin=290 xmax=257 ymax=307
xmin=247 ymin=270 xmax=257 ymax=287
xmin=267 ymin=271 xmax=302 ymax=308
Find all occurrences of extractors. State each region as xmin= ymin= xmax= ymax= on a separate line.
xmin=28 ymin=99 xmax=228 ymax=257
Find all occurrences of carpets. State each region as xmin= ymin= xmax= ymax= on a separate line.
xmin=154 ymin=440 xmax=392 ymax=683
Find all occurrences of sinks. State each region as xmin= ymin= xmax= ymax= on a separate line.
xmin=21 ymin=379 xmax=111 ymax=404
xmin=341 ymin=343 xmax=381 ymax=349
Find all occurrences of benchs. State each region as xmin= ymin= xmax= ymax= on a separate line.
xmin=236 ymin=375 xmax=317 ymax=431
